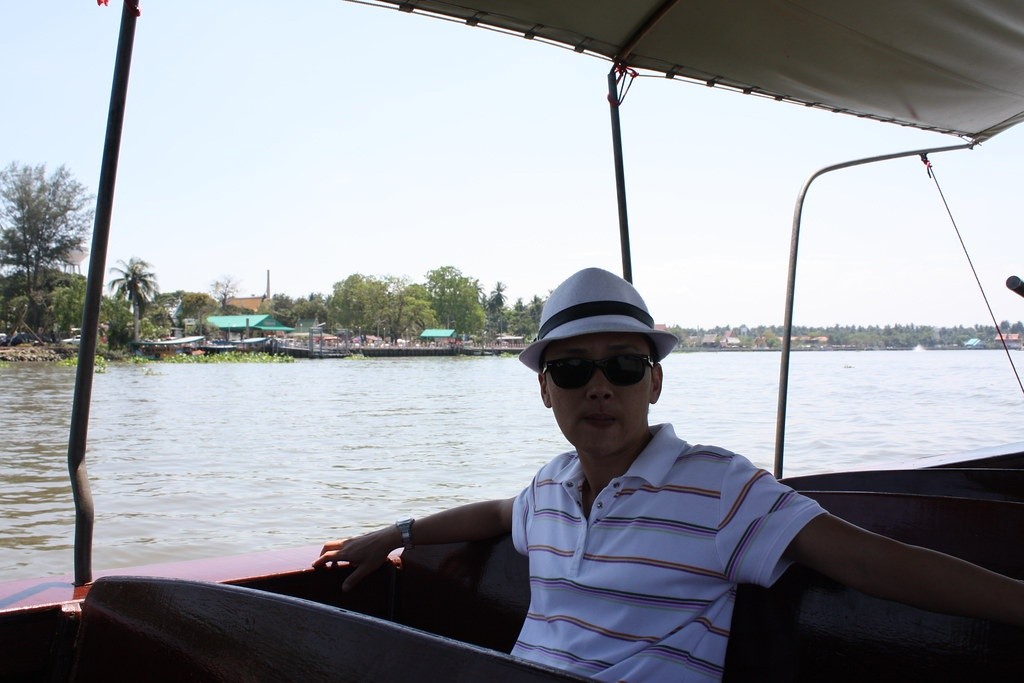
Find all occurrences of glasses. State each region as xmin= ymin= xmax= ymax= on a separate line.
xmin=543 ymin=354 xmax=653 ymax=389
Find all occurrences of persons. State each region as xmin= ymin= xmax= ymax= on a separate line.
xmin=312 ymin=268 xmax=1024 ymax=683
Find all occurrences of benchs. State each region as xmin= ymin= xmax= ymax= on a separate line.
xmin=67 ymin=468 xmax=1024 ymax=682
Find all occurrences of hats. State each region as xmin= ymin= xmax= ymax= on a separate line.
xmin=519 ymin=269 xmax=679 ymax=375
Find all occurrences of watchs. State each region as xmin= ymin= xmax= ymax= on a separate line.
xmin=396 ymin=519 xmax=415 ymax=550
